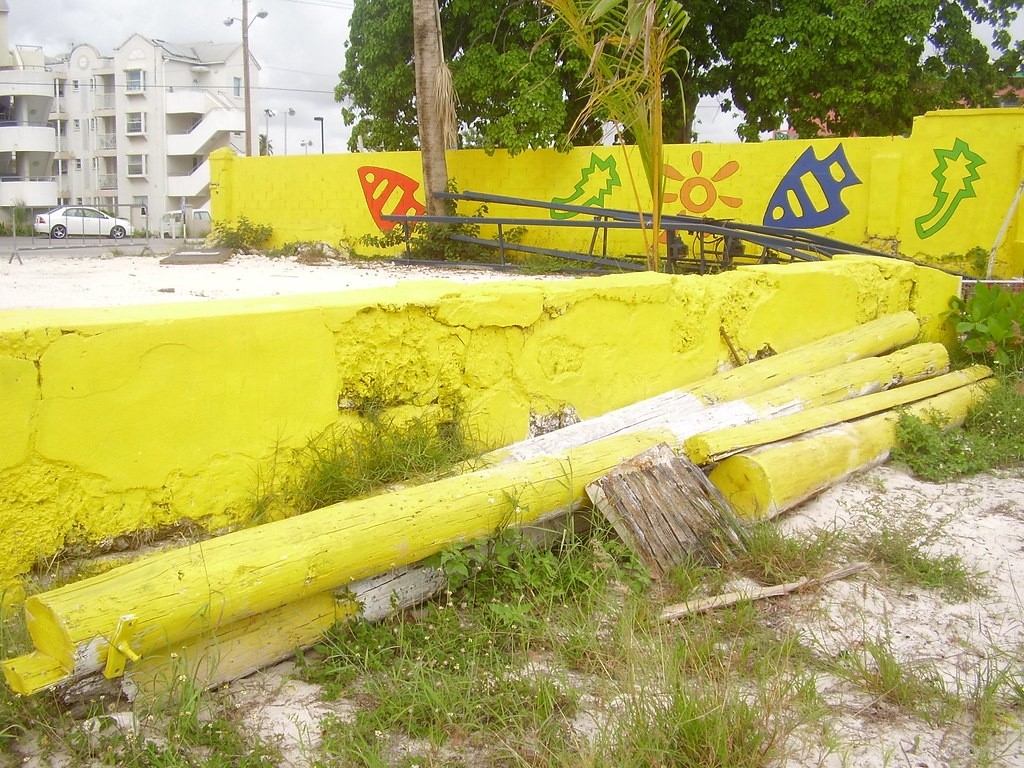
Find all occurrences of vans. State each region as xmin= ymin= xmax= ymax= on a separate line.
xmin=161 ymin=209 xmax=213 ymax=239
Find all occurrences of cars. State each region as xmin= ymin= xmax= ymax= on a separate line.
xmin=34 ymin=206 xmax=135 ymax=240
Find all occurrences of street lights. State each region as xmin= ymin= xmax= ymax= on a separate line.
xmin=314 ymin=117 xmax=325 ymax=154
xmin=285 ymin=107 xmax=296 ymax=154
xmin=223 ymin=0 xmax=269 ymax=159
